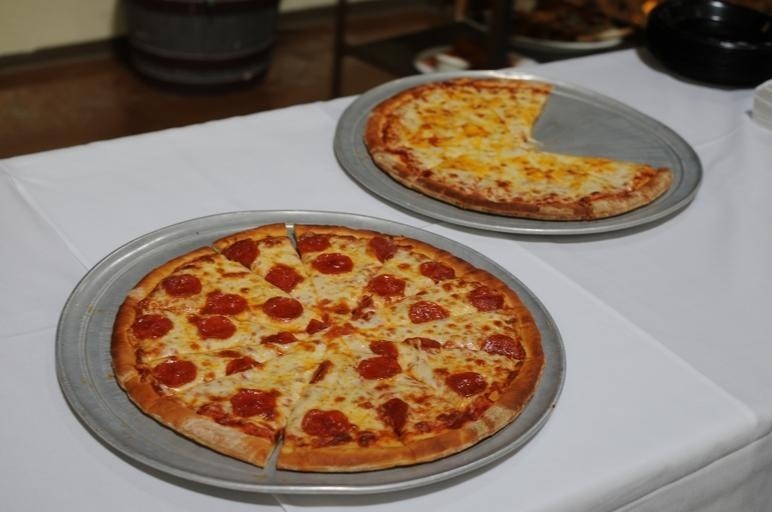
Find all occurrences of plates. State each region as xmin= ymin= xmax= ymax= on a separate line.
xmin=467 ymin=12 xmax=627 ymax=60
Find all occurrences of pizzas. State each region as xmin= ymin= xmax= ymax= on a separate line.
xmin=363 ymin=76 xmax=673 ymax=222
xmin=111 ymin=223 xmax=545 ymax=472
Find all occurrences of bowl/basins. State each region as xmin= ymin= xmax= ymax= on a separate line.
xmin=644 ymin=0 xmax=772 ymax=89
